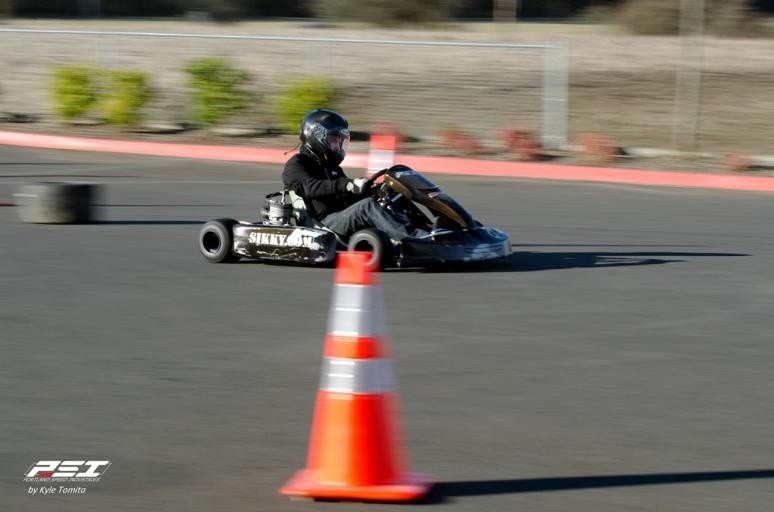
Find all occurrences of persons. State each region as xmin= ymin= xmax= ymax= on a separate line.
xmin=282 ymin=108 xmax=454 ymax=244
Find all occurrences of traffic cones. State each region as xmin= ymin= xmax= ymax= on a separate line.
xmin=270 ymin=248 xmax=441 ymax=508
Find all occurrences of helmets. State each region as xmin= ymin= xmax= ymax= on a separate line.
xmin=297 ymin=108 xmax=351 ymax=168
